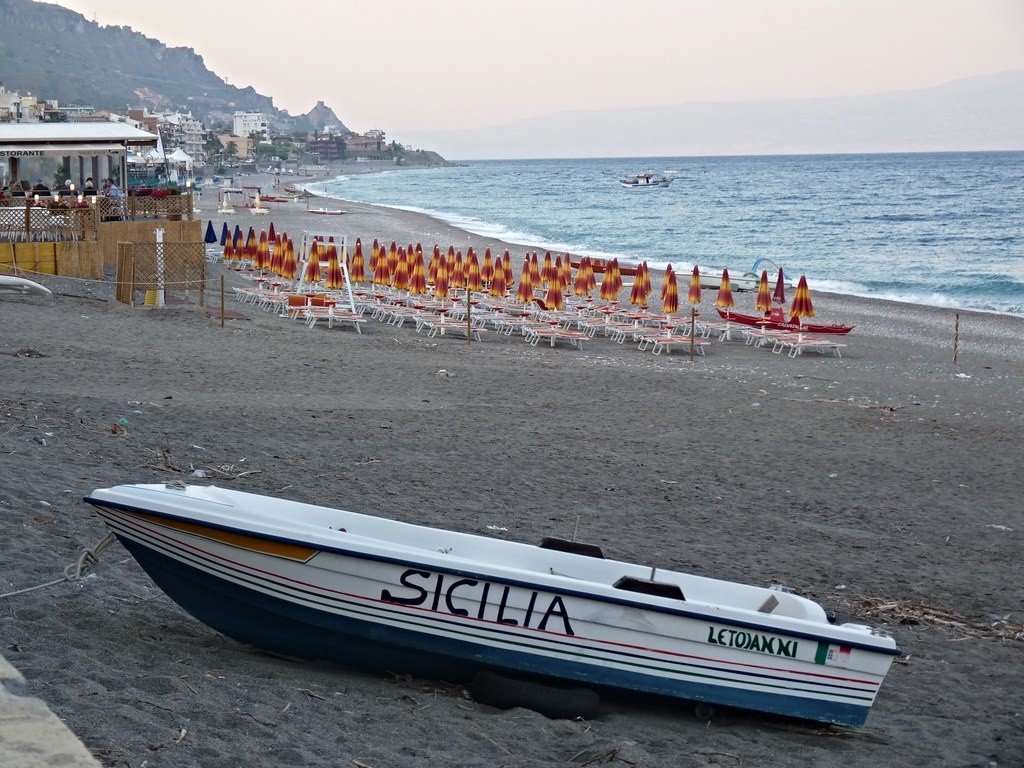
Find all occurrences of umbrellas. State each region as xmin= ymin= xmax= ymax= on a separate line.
xmin=629 ymin=261 xmax=652 ymax=306
xmin=516 ymin=250 xmax=623 ymax=320
xmin=220 ymin=221 xmax=367 ymax=294
xmin=204 ymin=219 xmax=217 ymax=244
xmin=687 ymin=264 xmax=702 ymax=304
xmin=715 ymin=268 xmax=734 ymax=342
xmin=754 ymin=269 xmax=772 ymax=334
xmin=368 ymin=237 xmax=514 ymax=336
xmin=772 ymin=265 xmax=816 ymax=355
xmin=659 ymin=264 xmax=679 ymax=353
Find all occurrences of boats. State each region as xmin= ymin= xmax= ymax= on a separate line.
xmin=743 ymin=272 xmax=793 ymax=291
xmin=306 ymin=208 xmax=347 ymax=215
xmin=269 ymin=198 xmax=288 ymax=202
xmin=620 ymin=169 xmax=673 ymax=188
xmin=570 ymin=258 xmax=649 ymax=276
xmin=249 ymin=194 xmax=276 ymax=201
xmin=674 ymin=273 xmax=757 ymax=292
xmin=716 ymin=307 xmax=857 ymax=337
xmin=242 ymin=184 xmax=262 ymax=189
xmin=83 ymin=480 xmax=903 ymax=732
xmin=0 ymin=273 xmax=51 ymax=296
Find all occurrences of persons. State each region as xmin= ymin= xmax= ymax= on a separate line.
xmin=1 ymin=177 xmax=123 ymax=222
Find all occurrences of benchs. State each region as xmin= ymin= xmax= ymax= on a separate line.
xmin=205 ymin=248 xmax=847 ymax=358
